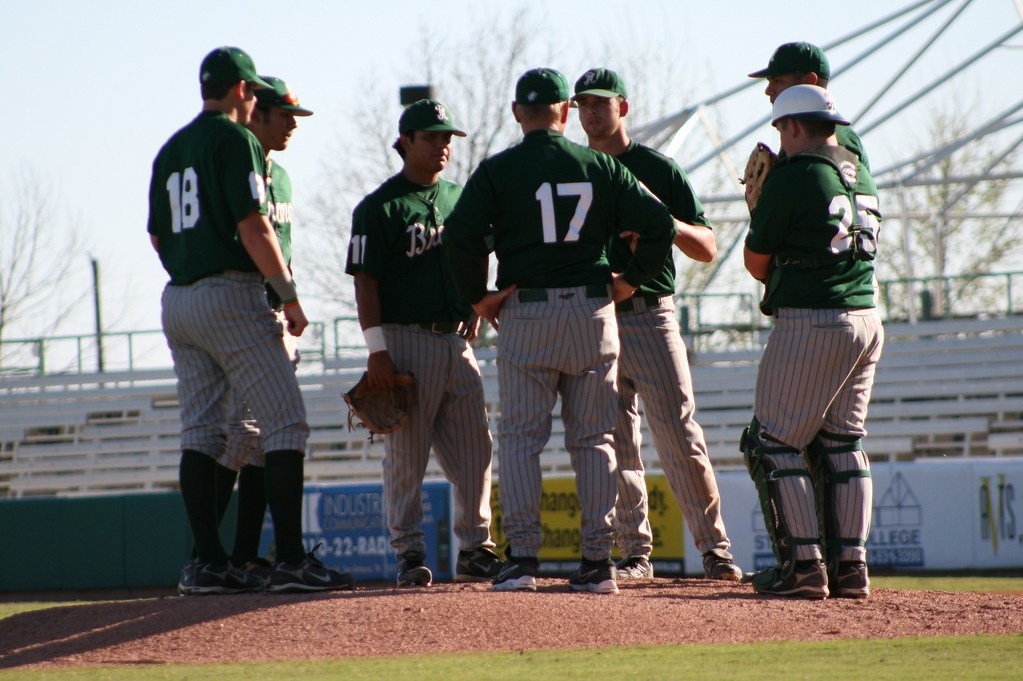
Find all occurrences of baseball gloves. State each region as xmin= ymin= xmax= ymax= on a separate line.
xmin=341 ymin=370 xmax=420 ymax=435
xmin=744 ymin=142 xmax=778 ymax=219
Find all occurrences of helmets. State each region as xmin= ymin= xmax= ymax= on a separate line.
xmin=771 ymin=83 xmax=851 ymax=125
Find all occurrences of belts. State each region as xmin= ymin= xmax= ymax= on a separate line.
xmin=615 ymin=293 xmax=659 ymax=311
xmin=518 ymin=284 xmax=608 ymax=302
xmin=166 ymin=269 xmax=222 ymax=286
xmin=422 ymin=321 xmax=460 ymax=334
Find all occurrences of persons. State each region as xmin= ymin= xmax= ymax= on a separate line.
xmin=567 ymin=68 xmax=742 ymax=582
xmin=744 ymin=42 xmax=886 ymax=600
xmin=345 ymin=99 xmax=502 ymax=591
xmin=148 ymin=45 xmax=352 ymax=595
xmin=442 ymin=67 xmax=675 ymax=596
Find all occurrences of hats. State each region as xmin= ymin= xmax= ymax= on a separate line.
xmin=570 ymin=67 xmax=628 ymax=98
xmin=748 ymin=40 xmax=831 ymax=81
xmin=200 ymin=46 xmax=277 ymax=91
xmin=254 ymin=76 xmax=314 ymax=116
xmin=516 ymin=67 xmax=578 ymax=108
xmin=400 ymin=98 xmax=468 ymax=138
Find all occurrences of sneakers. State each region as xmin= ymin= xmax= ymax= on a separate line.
xmin=192 ymin=556 xmax=271 ymax=596
xmin=397 ymin=550 xmax=433 ymax=587
xmin=490 ymin=556 xmax=538 ymax=590
xmin=700 ymin=548 xmax=743 ymax=581
xmin=177 ymin=557 xmax=203 ymax=597
xmin=455 ymin=546 xmax=506 ymax=583
xmin=614 ymin=556 xmax=654 ymax=580
xmin=233 ymin=556 xmax=277 ymax=588
xmin=269 ymin=553 xmax=354 ymax=592
xmin=568 ymin=558 xmax=620 ymax=596
xmin=750 ymin=558 xmax=830 ymax=600
xmin=826 ymin=559 xmax=870 ymax=598
